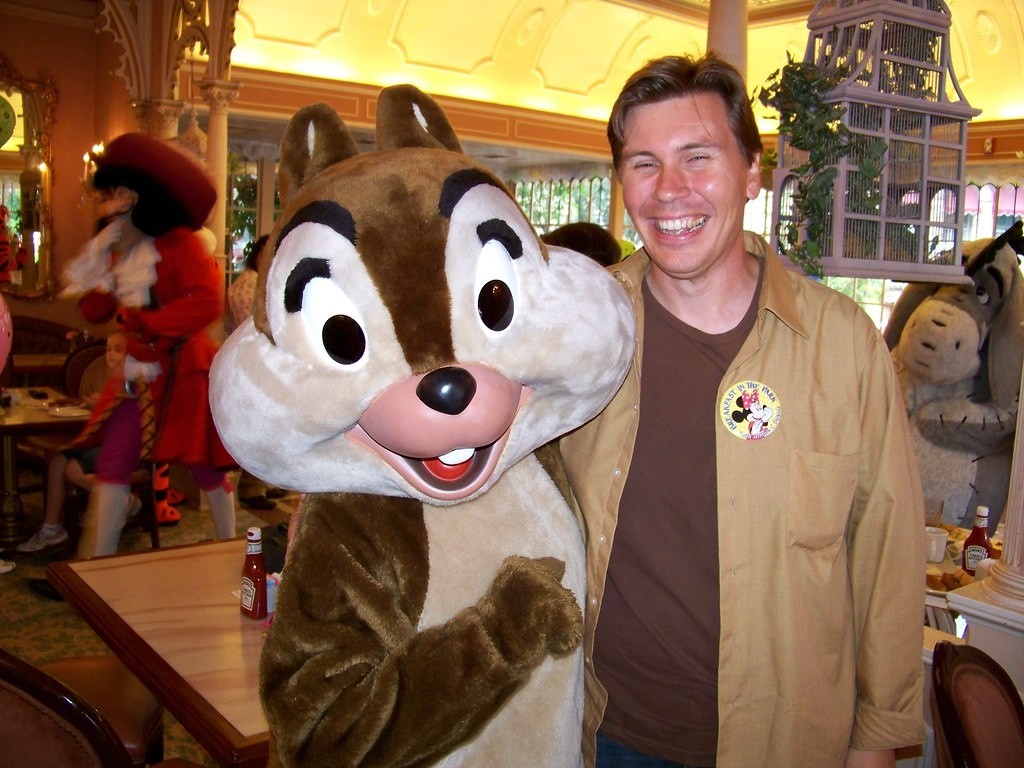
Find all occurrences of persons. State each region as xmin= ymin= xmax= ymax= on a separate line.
xmin=287 ymin=50 xmax=929 ymax=768
xmin=16 ymin=132 xmax=288 ymax=601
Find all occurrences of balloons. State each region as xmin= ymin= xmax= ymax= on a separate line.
xmin=0 ymin=293 xmax=12 ymax=373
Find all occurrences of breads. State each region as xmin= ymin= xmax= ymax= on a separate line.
xmin=926 ymin=569 xmax=973 ymax=592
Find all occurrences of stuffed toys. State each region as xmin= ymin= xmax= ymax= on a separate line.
xmin=883 ymin=239 xmax=1024 ymax=538
xmin=207 ymin=84 xmax=636 ymax=768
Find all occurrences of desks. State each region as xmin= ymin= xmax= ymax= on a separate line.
xmin=47 ymin=525 xmax=356 ymax=768
xmin=0 ymin=387 xmax=93 ymax=559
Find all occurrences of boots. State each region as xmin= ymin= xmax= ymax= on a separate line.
xmin=153 ymin=460 xmax=185 ymax=526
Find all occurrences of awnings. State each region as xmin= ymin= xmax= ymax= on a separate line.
xmin=901 ymin=190 xmax=919 ymax=206
xmin=992 ymin=185 xmax=1024 ymax=218
xmin=945 ymin=184 xmax=980 ymax=215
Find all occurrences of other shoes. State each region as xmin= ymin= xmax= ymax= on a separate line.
xmin=267 ymin=487 xmax=288 ymax=499
xmin=1 ymin=559 xmax=17 ymax=575
xmin=242 ymin=499 xmax=276 ymax=510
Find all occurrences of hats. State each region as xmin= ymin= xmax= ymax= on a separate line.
xmin=89 ymin=132 xmax=215 ymax=240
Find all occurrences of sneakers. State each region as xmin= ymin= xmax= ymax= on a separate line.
xmin=125 ymin=489 xmax=142 ymax=521
xmin=16 ymin=522 xmax=69 ymax=553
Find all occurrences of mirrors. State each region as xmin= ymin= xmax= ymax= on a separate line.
xmin=0 ymin=70 xmax=61 ymax=300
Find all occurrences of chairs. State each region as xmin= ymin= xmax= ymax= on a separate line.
xmin=929 ymin=639 xmax=1024 ymax=767
xmin=0 ymin=647 xmax=207 ymax=767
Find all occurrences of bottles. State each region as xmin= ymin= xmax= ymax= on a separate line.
xmin=240 ymin=526 xmax=268 ymax=620
xmin=990 ymin=523 xmax=1004 ymax=560
xmin=961 ymin=505 xmax=994 ymax=577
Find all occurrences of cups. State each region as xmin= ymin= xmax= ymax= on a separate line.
xmin=925 ymin=526 xmax=949 ymax=563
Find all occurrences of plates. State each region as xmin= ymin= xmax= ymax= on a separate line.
xmin=924 ymin=586 xmax=948 ymax=597
xmin=946 ymin=528 xmax=987 ymax=544
xmin=48 ymin=407 xmax=90 ymax=416
